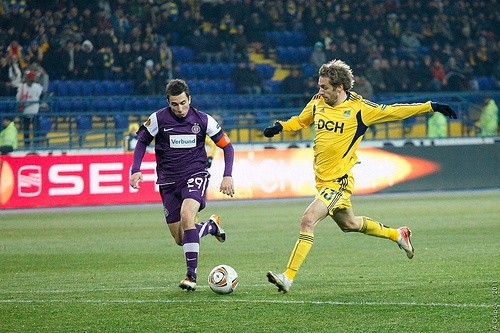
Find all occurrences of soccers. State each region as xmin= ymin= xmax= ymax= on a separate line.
xmin=207 ymin=262 xmax=240 ymax=295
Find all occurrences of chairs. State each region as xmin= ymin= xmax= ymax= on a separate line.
xmin=0 ymin=29 xmax=500 ymax=130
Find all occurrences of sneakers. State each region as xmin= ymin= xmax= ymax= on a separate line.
xmin=395 ymin=226 xmax=414 ymax=259
xmin=266 ymin=271 xmax=294 ymax=292
xmin=209 ymin=214 xmax=225 ymax=243
xmin=179 ymin=276 xmax=196 ymax=291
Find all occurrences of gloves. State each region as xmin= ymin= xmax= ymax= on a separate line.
xmin=431 ymin=101 xmax=458 ymax=119
xmin=264 ymin=122 xmax=283 ymax=138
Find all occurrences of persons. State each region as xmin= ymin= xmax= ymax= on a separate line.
xmin=130 ymin=79 xmax=235 ymax=291
xmin=0 ymin=0 xmax=500 ymax=156
xmin=263 ymin=59 xmax=458 ymax=294
